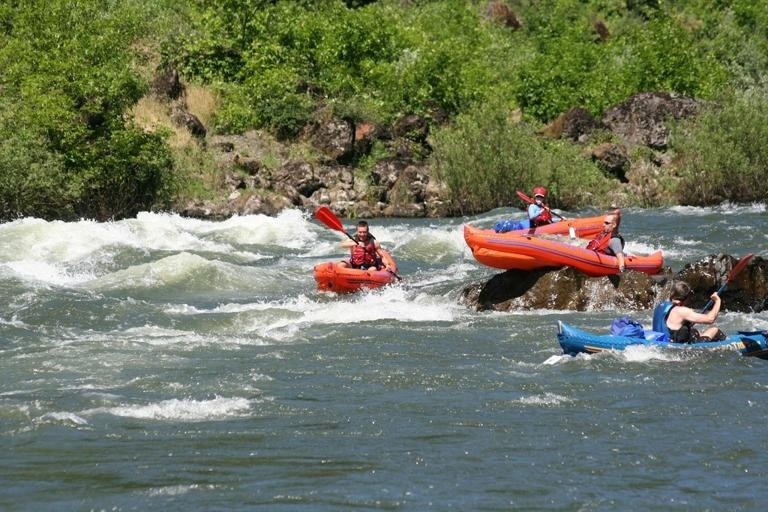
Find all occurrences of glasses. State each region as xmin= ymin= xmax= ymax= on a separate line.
xmin=604 ymin=221 xmax=613 ymax=225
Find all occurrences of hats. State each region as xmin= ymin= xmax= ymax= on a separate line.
xmin=532 ymin=186 xmax=547 ymax=198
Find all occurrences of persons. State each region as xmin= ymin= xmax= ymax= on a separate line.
xmin=586 ymin=214 xmax=625 ymax=273
xmin=340 ymin=221 xmax=391 ymax=271
xmin=653 ymin=281 xmax=726 ymax=342
xmin=528 ymin=186 xmax=566 ymax=228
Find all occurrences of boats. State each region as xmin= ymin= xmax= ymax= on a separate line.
xmin=555 ymin=319 xmax=768 ymax=356
xmin=312 ymin=248 xmax=397 ymax=294
xmin=463 ymin=209 xmax=663 ymax=275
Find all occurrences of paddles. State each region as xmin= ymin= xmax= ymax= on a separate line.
xmin=316 ymin=208 xmax=402 ymax=281
xmin=688 ymin=253 xmax=754 ymax=328
xmin=517 ymin=191 xmax=565 ymax=220
